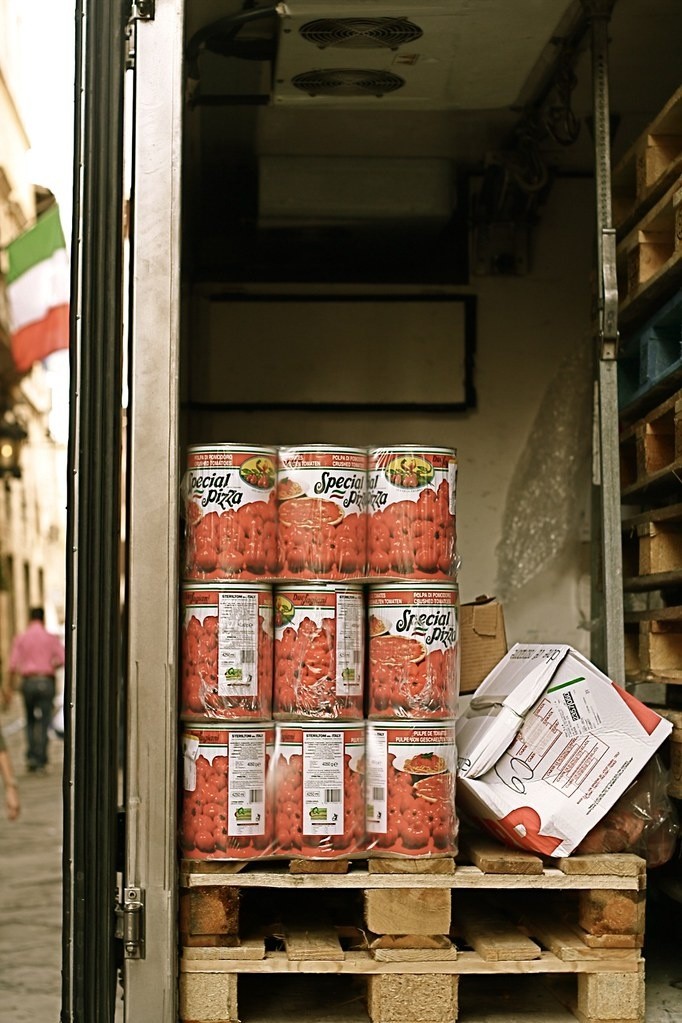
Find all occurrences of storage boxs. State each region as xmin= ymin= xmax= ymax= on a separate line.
xmin=455 ymin=643 xmax=673 ymax=855
xmin=460 ymin=594 xmax=508 ymax=695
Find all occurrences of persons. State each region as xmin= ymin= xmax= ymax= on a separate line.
xmin=2 ymin=605 xmax=65 ymax=773
xmin=0 ymin=728 xmax=21 ymax=822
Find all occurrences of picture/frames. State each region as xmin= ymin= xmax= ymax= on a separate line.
xmin=197 ymin=292 xmax=481 ymax=412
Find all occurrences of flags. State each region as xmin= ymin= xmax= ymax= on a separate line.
xmin=0 ymin=206 xmax=71 ymax=372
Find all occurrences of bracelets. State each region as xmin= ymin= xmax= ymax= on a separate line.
xmin=4 ymin=778 xmax=19 ymax=788
